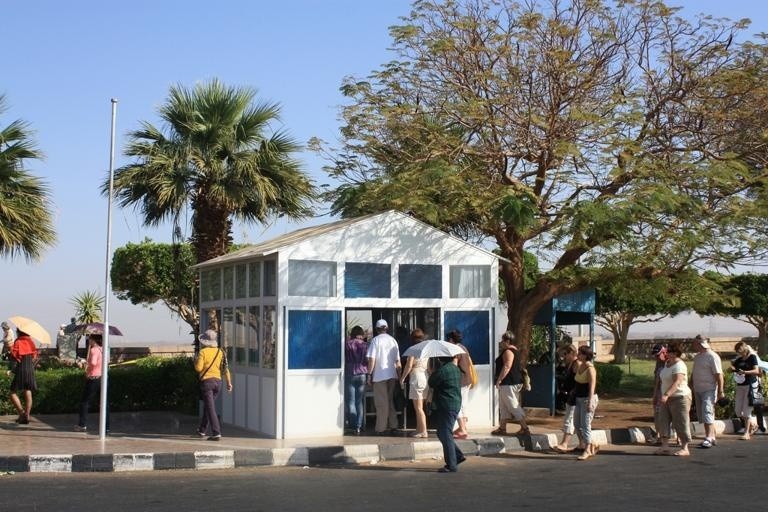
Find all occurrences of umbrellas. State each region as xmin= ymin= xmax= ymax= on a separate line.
xmin=68 ymin=321 xmax=122 ymax=335
xmin=8 ymin=316 xmax=52 ymax=344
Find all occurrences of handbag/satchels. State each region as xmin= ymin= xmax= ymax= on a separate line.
xmin=394 ymin=381 xmax=406 ymax=412
xmin=467 ymin=353 xmax=476 ymax=389
xmin=568 ymin=391 xmax=576 ymax=406
xmin=427 ymin=388 xmax=434 ymax=403
xmin=560 ymin=392 xmax=568 ymax=402
xmin=221 ymin=354 xmax=228 ymax=375
xmin=749 ymin=386 xmax=765 ymax=406
xmin=520 ymin=368 xmax=531 ymax=392
xmin=192 ymin=380 xmax=203 ymax=400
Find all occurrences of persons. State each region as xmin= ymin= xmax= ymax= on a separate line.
xmin=5 ymin=328 xmax=37 ymax=424
xmin=193 ymin=330 xmax=232 ymax=440
xmin=56 ymin=323 xmax=67 ymax=358
xmin=0 ymin=321 xmax=14 ymax=361
xmin=651 ymin=334 xmax=767 ymax=456
xmin=74 ymin=334 xmax=110 ymax=433
xmin=346 ymin=317 xmax=602 ymax=474
xmin=65 ymin=318 xmax=83 ymax=358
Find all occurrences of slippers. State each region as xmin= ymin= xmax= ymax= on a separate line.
xmin=655 ymin=448 xmax=690 ymax=456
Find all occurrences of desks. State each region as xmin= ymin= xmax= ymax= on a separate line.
xmin=363 ymin=382 xmax=408 ymax=430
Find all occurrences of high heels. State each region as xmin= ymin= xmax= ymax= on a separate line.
xmin=453 ymin=429 xmax=467 ymax=439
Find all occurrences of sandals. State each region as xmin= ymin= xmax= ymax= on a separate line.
xmin=516 ymin=427 xmax=530 ymax=435
xmin=492 ymin=427 xmax=507 ymax=435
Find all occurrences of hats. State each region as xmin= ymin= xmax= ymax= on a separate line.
xmin=377 ymin=319 xmax=388 ymax=328
xmin=734 ymin=368 xmax=746 ymax=384
xmin=198 ymin=330 xmax=217 ymax=347
xmin=651 ymin=345 xmax=667 ymax=355
xmin=696 ymin=335 xmax=711 ymax=349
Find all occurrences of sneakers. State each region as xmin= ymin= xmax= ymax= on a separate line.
xmin=737 ymin=426 xmax=768 ymax=439
xmin=554 ymin=444 xmax=599 ymax=460
xmin=73 ymin=425 xmax=86 ymax=432
xmin=438 ymin=456 xmax=466 ymax=473
xmin=701 ymin=436 xmax=717 ymax=447
xmin=16 ymin=414 xmax=29 ymax=423
xmin=352 ymin=428 xmax=428 ymax=438
xmin=196 ymin=428 xmax=222 ymax=441
